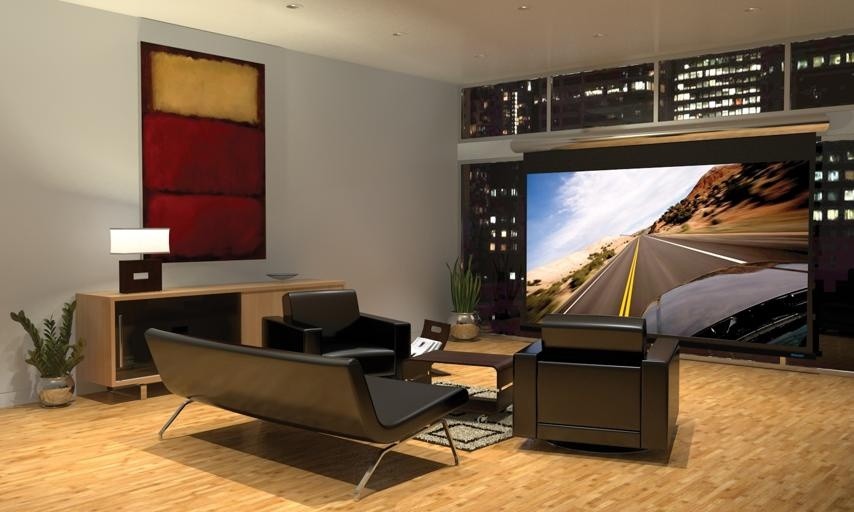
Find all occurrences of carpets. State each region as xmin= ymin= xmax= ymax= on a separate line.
xmin=412 ymin=381 xmax=515 ymax=452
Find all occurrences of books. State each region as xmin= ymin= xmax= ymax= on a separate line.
xmin=411 ymin=336 xmax=442 ymax=358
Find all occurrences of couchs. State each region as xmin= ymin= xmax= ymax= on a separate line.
xmin=143 ymin=328 xmax=469 ymax=502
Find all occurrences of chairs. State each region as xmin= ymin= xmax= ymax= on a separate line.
xmin=262 ymin=289 xmax=411 ymax=380
xmin=513 ymin=314 xmax=680 ymax=457
xmin=410 ymin=320 xmax=451 ymax=377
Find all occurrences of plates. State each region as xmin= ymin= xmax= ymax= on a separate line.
xmin=266 ymin=274 xmax=298 ymax=281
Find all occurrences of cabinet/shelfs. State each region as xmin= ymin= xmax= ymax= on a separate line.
xmin=76 ymin=278 xmax=345 ymax=400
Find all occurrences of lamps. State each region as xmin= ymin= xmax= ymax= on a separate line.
xmin=110 ymin=228 xmax=170 ymax=293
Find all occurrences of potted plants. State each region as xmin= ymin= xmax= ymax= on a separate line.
xmin=10 ymin=301 xmax=87 ymax=408
xmin=446 ymin=255 xmax=481 ymax=341
